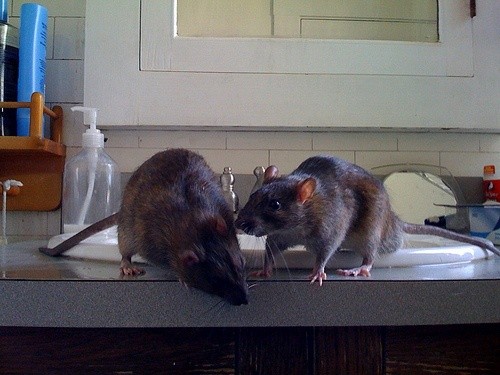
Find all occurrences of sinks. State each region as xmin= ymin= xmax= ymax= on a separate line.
xmin=47 ymin=233 xmax=494 ymax=269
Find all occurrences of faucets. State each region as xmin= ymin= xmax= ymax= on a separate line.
xmin=251 ymin=166 xmax=265 ymax=193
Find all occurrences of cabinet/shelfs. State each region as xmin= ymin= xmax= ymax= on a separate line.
xmin=0 ymin=324 xmax=500 ymax=375
